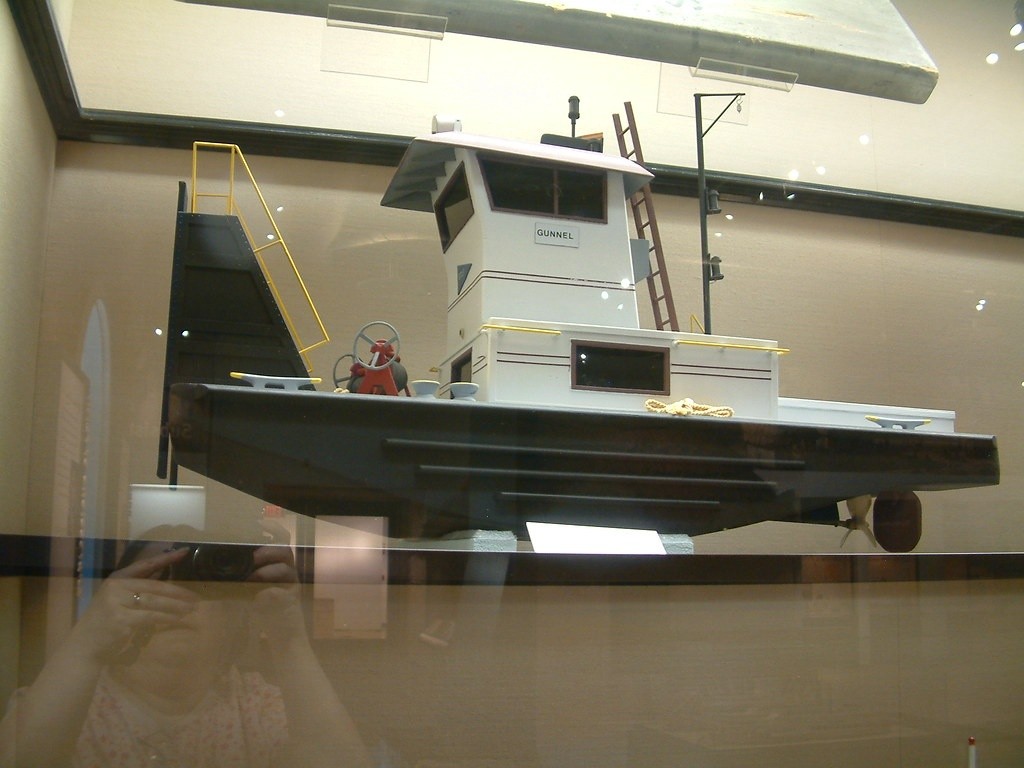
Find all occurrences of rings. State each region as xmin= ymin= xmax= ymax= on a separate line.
xmin=131 ymin=592 xmax=142 ymax=609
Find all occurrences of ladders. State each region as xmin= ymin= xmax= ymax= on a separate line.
xmin=607 ymin=101 xmax=686 ymax=332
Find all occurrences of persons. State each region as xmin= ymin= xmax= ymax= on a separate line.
xmin=0 ymin=516 xmax=377 ymax=768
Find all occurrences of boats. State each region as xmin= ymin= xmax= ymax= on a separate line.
xmin=167 ymin=93 xmax=1000 ymax=537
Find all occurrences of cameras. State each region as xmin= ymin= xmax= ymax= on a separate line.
xmin=159 ymin=532 xmax=259 ymax=606
